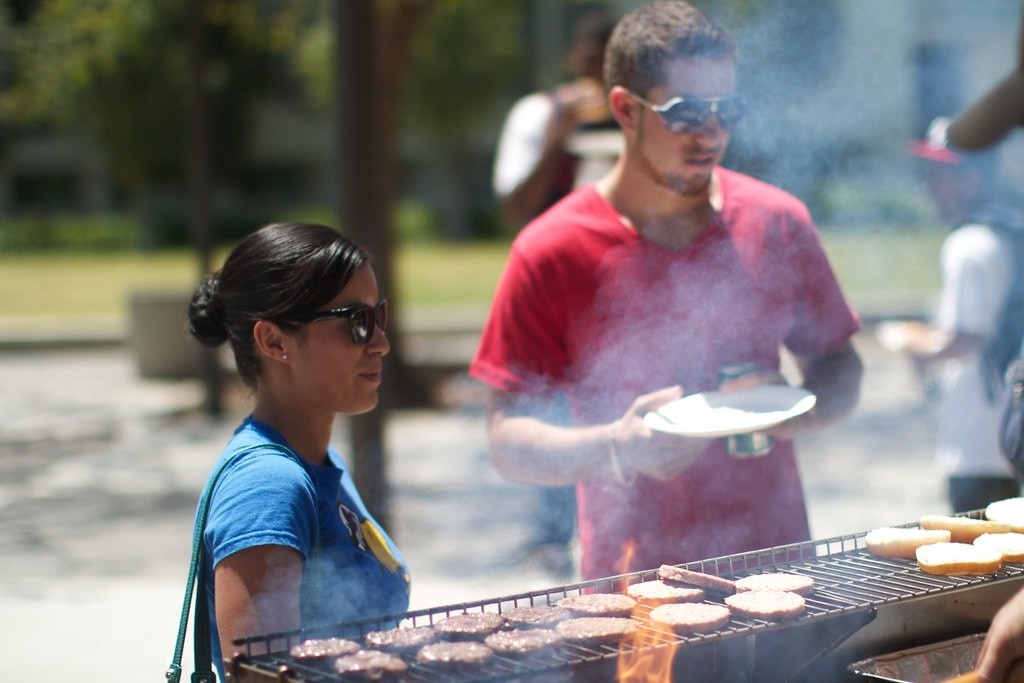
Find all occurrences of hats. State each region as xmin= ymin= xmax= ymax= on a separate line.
xmin=905 ymin=115 xmax=994 ymax=168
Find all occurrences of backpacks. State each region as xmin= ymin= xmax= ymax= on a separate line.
xmin=941 ymin=209 xmax=1024 ymax=472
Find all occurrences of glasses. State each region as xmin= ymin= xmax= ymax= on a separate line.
xmin=631 ymin=93 xmax=747 ymax=135
xmin=305 ymin=299 xmax=388 ymax=345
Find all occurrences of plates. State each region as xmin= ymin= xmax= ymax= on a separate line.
xmin=644 ymin=385 xmax=817 ymax=438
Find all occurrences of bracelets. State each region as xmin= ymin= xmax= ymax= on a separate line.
xmin=607 ymin=418 xmax=637 ymax=489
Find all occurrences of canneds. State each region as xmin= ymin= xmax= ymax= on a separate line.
xmin=717 ymin=364 xmax=774 ymax=458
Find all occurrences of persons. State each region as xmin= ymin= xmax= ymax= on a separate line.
xmin=469 ymin=0 xmax=862 ymax=593
xmin=166 ymin=221 xmax=410 ymax=683
xmin=872 ymin=37 xmax=1024 ymax=682
